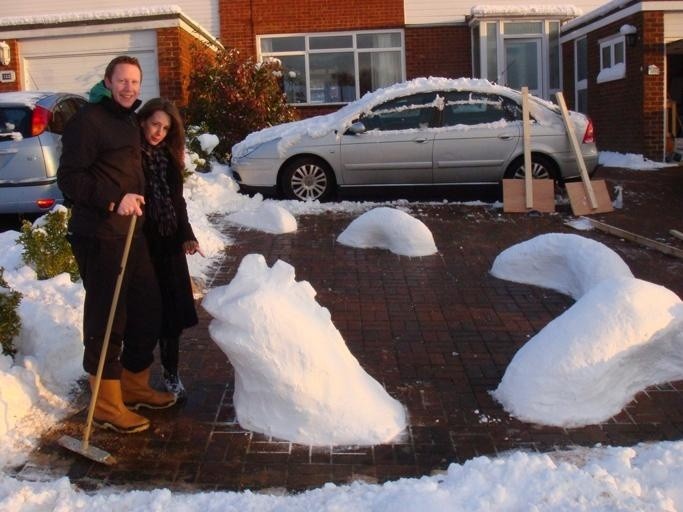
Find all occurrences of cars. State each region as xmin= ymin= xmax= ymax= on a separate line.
xmin=0 ymin=90 xmax=91 ymax=216
xmin=230 ymin=75 xmax=599 ymax=202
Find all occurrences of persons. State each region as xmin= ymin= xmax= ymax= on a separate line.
xmin=56 ymin=55 xmax=177 ymax=437
xmin=137 ymin=93 xmax=208 ymax=403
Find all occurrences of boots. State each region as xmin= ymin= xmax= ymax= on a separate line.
xmin=88 ymin=362 xmax=185 ymax=433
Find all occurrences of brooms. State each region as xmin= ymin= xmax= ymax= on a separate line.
xmin=55 ymin=195 xmax=143 ymax=467
